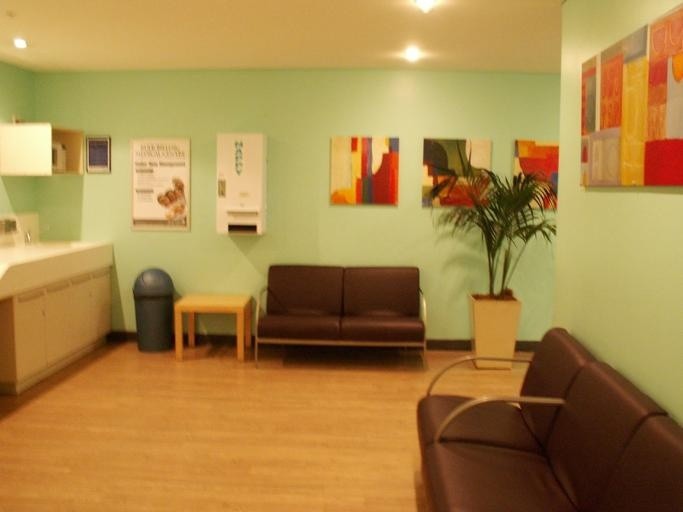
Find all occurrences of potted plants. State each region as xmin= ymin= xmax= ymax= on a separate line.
xmin=429 ymin=140 xmax=556 ymax=369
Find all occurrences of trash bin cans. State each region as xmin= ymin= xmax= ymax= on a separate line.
xmin=134 ymin=269 xmax=175 ymax=352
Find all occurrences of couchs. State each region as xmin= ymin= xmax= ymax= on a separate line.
xmin=416 ymin=326 xmax=683 ymax=511
xmin=254 ymin=265 xmax=428 ymax=370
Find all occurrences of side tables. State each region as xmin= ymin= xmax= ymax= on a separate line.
xmin=173 ymin=295 xmax=252 ymax=362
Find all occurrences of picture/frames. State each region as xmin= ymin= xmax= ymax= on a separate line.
xmin=421 ymin=138 xmax=492 ymax=208
xmin=85 ymin=137 xmax=111 ymax=174
xmin=329 ymin=134 xmax=399 ymax=206
xmin=130 ymin=138 xmax=189 ymax=231
xmin=513 ymin=139 xmax=559 ymax=209
xmin=579 ymin=3 xmax=682 ymax=188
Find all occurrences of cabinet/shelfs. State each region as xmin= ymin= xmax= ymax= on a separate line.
xmin=0 ymin=267 xmax=110 ymax=396
